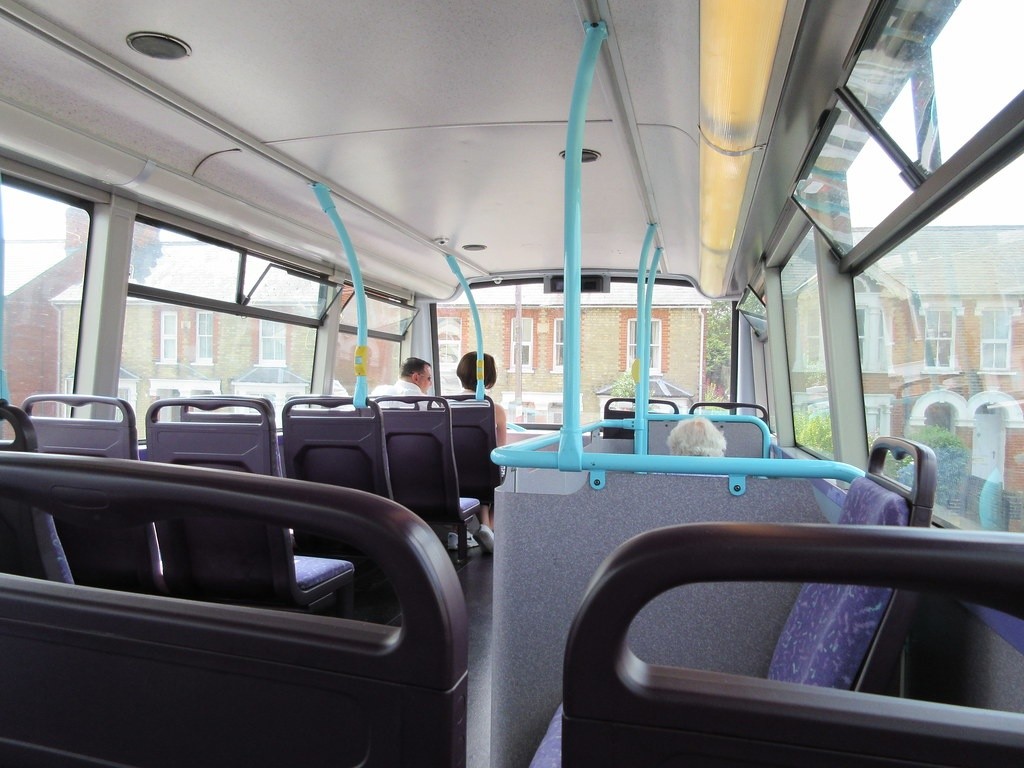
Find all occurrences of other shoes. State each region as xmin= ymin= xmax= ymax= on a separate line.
xmin=447 ymin=533 xmax=478 ymax=551
xmin=472 ymin=524 xmax=495 ymax=555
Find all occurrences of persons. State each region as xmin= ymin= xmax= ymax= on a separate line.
xmin=668 ymin=419 xmax=727 ymax=457
xmin=370 ymin=357 xmax=479 ymax=550
xmin=456 ymin=352 xmax=506 ymax=552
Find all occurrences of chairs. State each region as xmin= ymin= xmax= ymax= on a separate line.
xmin=23 ymin=392 xmax=172 ymax=599
xmin=146 ymin=396 xmax=355 ymax=619
xmin=690 ymin=402 xmax=770 ymax=424
xmin=524 ymin=435 xmax=940 ymax=768
xmin=438 ymin=393 xmax=502 ymax=506
xmin=283 ymin=393 xmax=393 ymax=501
xmin=374 ymin=394 xmax=482 ymax=562
xmin=0 ymin=450 xmax=465 ymax=768
xmin=557 ymin=520 xmax=1024 ymax=768
xmin=604 ymin=396 xmax=679 ymax=439
xmin=0 ymin=398 xmax=76 ymax=586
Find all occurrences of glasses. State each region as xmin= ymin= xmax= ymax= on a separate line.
xmin=419 ymin=374 xmax=432 ymax=380
